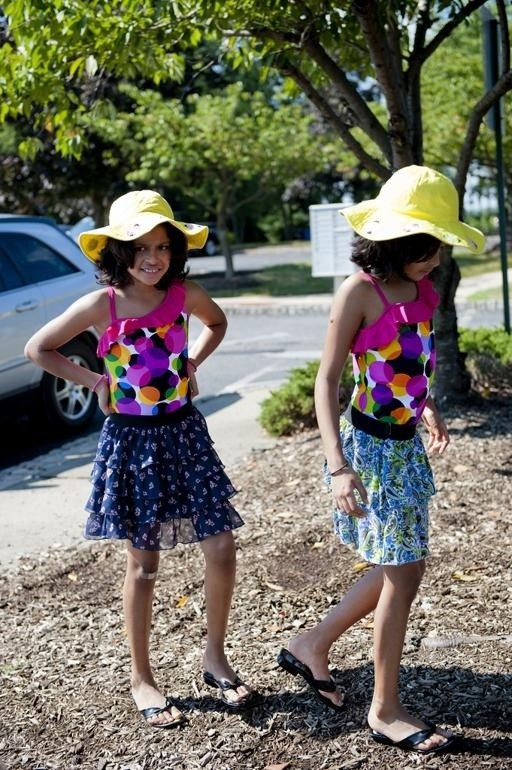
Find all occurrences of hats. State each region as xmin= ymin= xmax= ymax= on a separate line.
xmin=77 ymin=188 xmax=209 ymax=269
xmin=342 ymin=162 xmax=487 ymax=257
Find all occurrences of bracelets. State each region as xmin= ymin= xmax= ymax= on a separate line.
xmin=327 ymin=460 xmax=349 ymax=476
xmin=92 ymin=374 xmax=106 ymax=392
xmin=188 ymin=359 xmax=198 ymax=373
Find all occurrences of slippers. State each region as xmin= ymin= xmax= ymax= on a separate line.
xmin=199 ymin=666 xmax=257 ymax=709
xmin=277 ymin=646 xmax=346 ymax=710
xmin=131 ymin=684 xmax=185 ymax=728
xmin=365 ymin=713 xmax=458 ymax=752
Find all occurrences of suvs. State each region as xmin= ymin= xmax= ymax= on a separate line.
xmin=0 ymin=210 xmax=122 ymax=433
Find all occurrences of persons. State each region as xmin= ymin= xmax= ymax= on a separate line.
xmin=276 ymin=164 xmax=485 ymax=755
xmin=23 ymin=189 xmax=258 ymax=731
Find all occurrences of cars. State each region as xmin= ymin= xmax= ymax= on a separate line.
xmin=185 ymin=222 xmax=221 ymax=258
